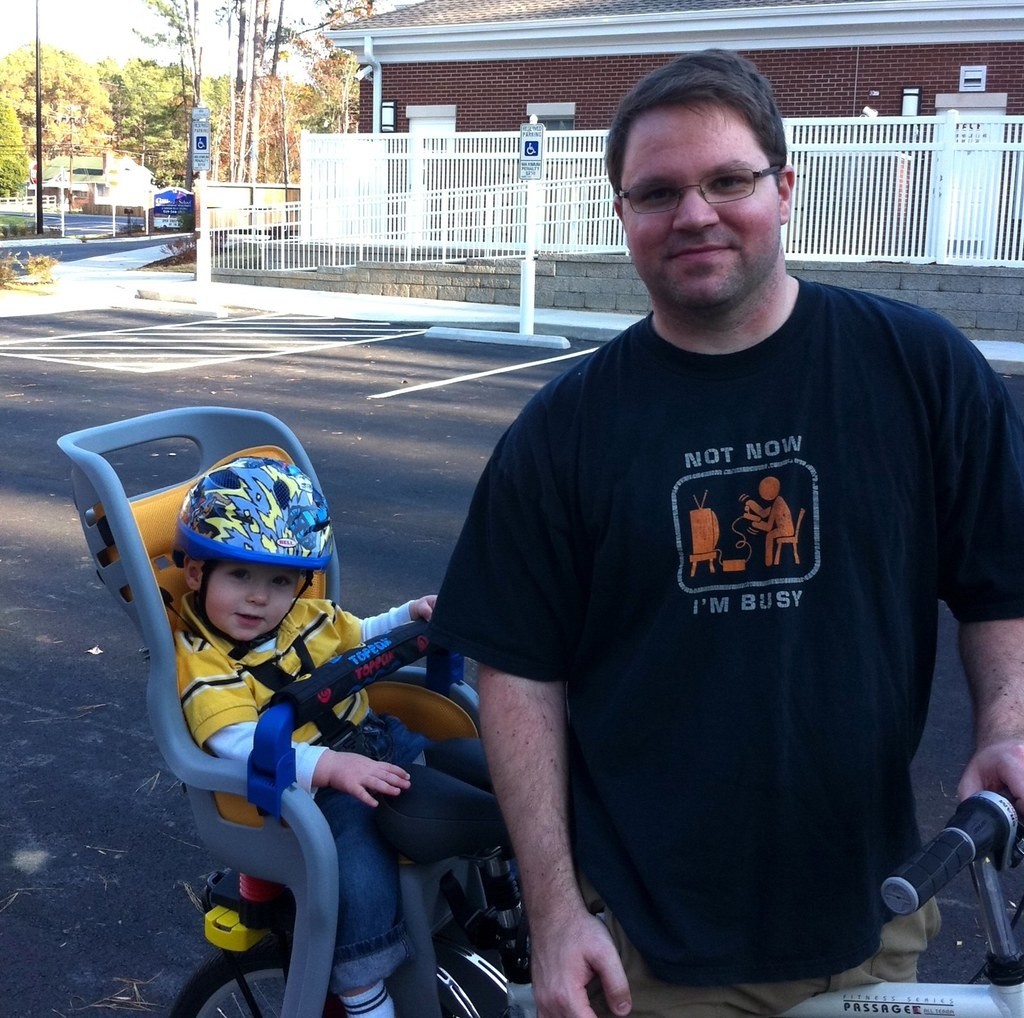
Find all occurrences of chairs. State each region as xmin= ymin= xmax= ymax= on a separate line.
xmin=57 ymin=404 xmax=481 ymax=1018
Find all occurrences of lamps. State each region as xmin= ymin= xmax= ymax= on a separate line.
xmin=381 ymin=99 xmax=397 ymax=132
xmin=902 ymin=85 xmax=922 ymax=116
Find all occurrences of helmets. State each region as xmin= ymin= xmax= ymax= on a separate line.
xmin=175 ymin=458 xmax=333 ymax=569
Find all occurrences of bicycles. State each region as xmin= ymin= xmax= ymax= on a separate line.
xmin=163 ymin=759 xmax=1024 ymax=1018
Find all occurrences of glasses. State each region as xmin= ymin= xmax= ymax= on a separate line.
xmin=619 ymin=165 xmax=780 ymax=213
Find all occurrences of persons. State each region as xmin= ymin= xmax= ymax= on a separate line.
xmin=173 ymin=456 xmax=488 ymax=1018
xmin=431 ymin=50 xmax=1024 ymax=1017
